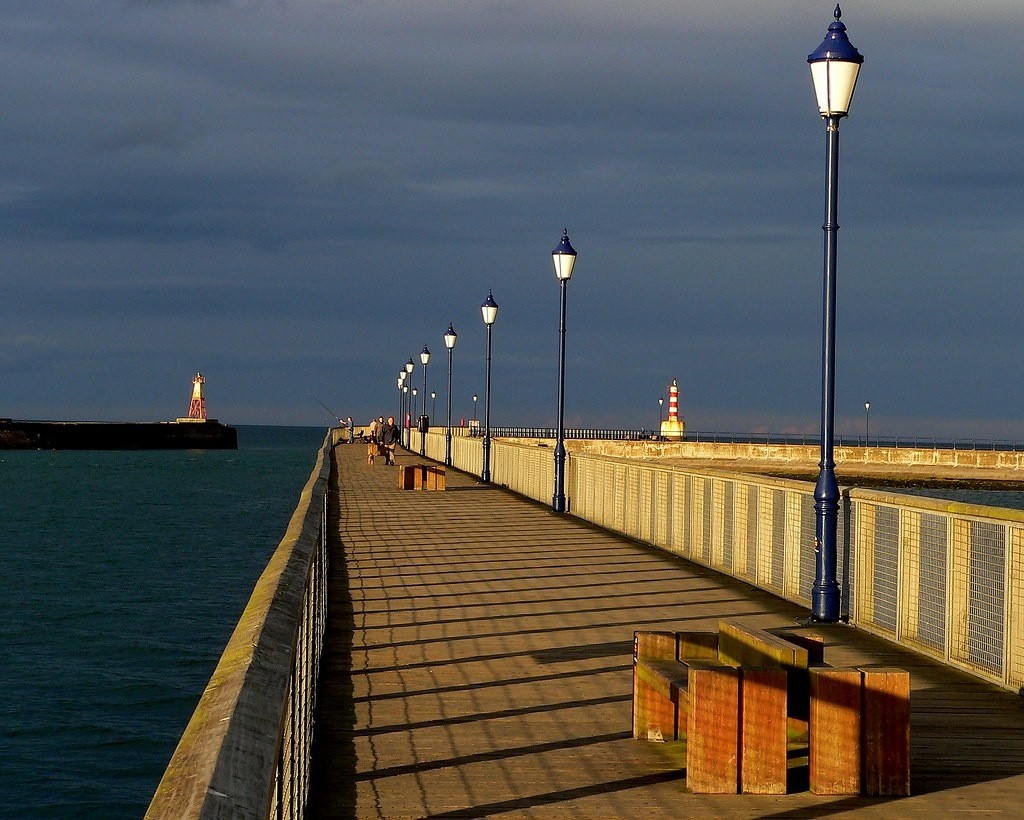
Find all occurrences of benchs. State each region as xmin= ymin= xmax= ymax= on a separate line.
xmin=631 ymin=618 xmax=792 ymax=796
xmin=728 ymin=616 xmax=910 ymax=796
xmin=398 ymin=460 xmax=446 ymax=492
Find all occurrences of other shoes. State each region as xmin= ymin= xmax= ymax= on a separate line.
xmin=390 ymin=462 xmax=394 ymax=465
xmin=385 ymin=462 xmax=388 ymax=465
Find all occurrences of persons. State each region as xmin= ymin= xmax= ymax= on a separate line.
xmin=380 ymin=416 xmax=400 ymax=466
xmin=374 ymin=415 xmax=386 ymax=451
xmin=369 ymin=418 xmax=377 ymax=438
xmin=341 ymin=417 xmax=354 ymax=444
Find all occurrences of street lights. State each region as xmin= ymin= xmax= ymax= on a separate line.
xmin=444 ymin=321 xmax=457 ymax=466
xmin=430 ymin=390 xmax=436 ymax=426
xmin=863 ymin=400 xmax=870 ymax=445
xmin=479 ymin=287 xmax=499 ymax=483
xmin=397 ymin=356 xmax=417 ymax=446
xmin=551 ymin=226 xmax=577 ymax=513
xmin=806 ymin=2 xmax=865 ymax=622
xmin=657 ymin=397 xmax=664 ymax=440
xmin=418 ymin=344 xmax=431 ymax=457
xmin=472 ymin=394 xmax=477 ymax=420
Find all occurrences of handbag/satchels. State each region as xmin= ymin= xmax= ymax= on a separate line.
xmin=380 ymin=444 xmax=386 ymax=456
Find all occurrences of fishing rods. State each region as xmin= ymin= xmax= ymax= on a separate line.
xmin=307 ymin=392 xmax=339 ymax=420
